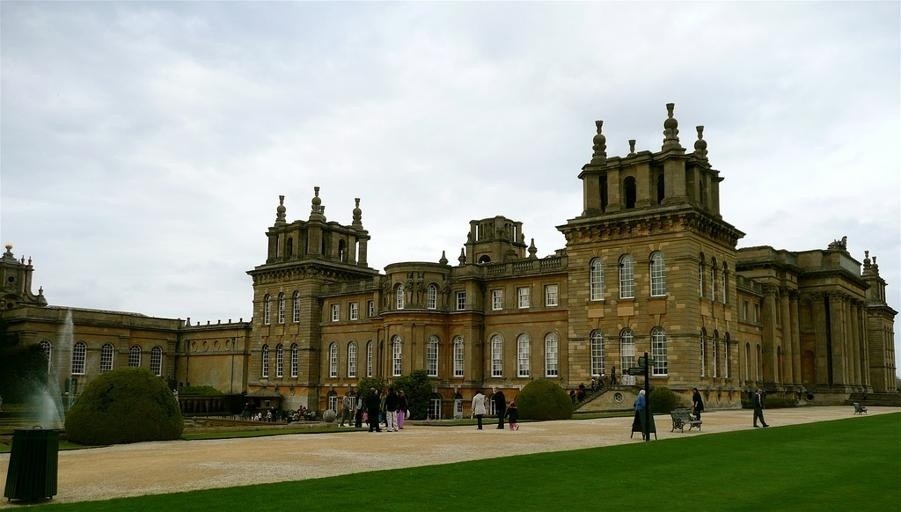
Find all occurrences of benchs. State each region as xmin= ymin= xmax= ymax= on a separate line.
xmin=670 ymin=410 xmax=702 ymax=433
xmin=853 ymin=402 xmax=869 ymax=416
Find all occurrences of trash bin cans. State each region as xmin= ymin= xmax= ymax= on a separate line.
xmin=5 ymin=426 xmax=61 ymax=503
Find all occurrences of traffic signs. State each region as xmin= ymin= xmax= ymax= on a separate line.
xmin=627 ymin=357 xmax=655 ymax=376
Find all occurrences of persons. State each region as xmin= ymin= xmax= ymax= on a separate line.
xmin=336 ymin=385 xmax=410 ymax=433
xmin=690 ymin=387 xmax=704 ymax=427
xmin=568 ymin=365 xmax=618 ymax=406
xmin=470 ymin=390 xmax=488 ymax=431
xmin=629 ymin=389 xmax=646 ymax=441
xmin=502 ymin=399 xmax=519 ymax=432
xmin=752 ymin=388 xmax=769 ymax=428
xmin=489 ymin=386 xmax=507 ymax=430
xmin=240 ymin=405 xmax=316 ymax=424
